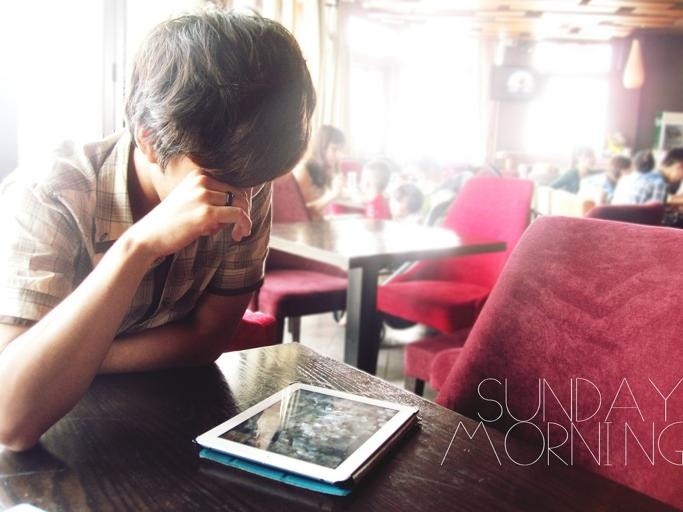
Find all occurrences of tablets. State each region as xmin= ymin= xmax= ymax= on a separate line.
xmin=193 ymin=380 xmax=421 ymax=487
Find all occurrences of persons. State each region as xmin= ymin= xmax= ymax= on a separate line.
xmin=0 ymin=5 xmax=318 ymax=453
xmin=297 ymin=127 xmax=683 ymax=224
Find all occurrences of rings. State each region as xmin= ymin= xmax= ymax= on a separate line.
xmin=226 ymin=191 xmax=234 ymax=206
